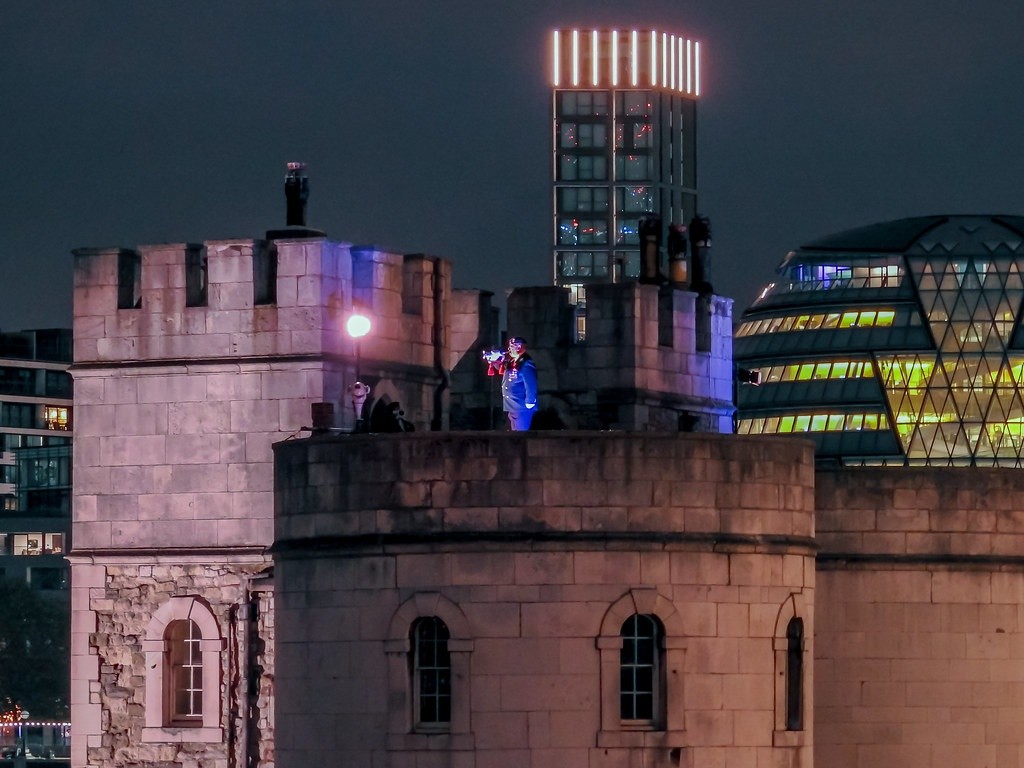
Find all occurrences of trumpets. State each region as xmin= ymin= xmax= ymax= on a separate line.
xmin=483 ymin=351 xmax=510 ymax=360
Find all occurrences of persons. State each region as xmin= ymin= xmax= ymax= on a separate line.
xmin=487 ymin=337 xmax=538 ymax=431
